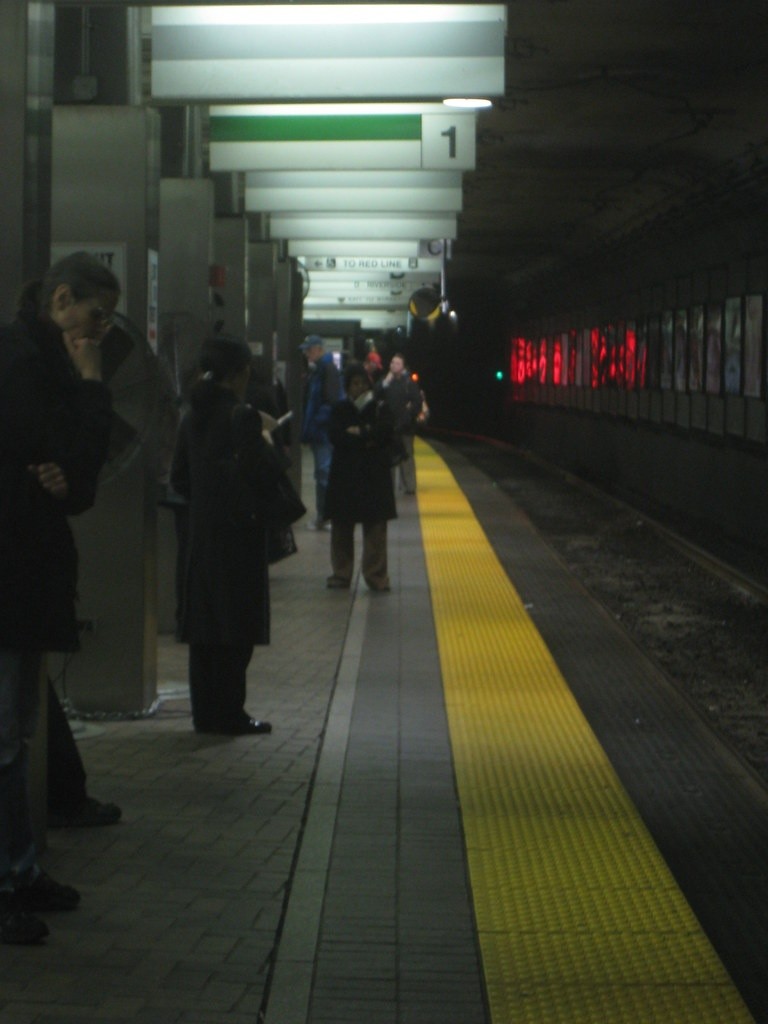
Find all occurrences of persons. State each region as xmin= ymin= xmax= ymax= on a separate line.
xmin=158 ymin=333 xmax=307 ymax=733
xmin=322 ymin=371 xmax=398 ymax=592
xmin=297 ymin=334 xmax=345 ymax=532
xmin=373 ymin=355 xmax=422 ymax=495
xmin=0 ymin=250 xmax=121 ymax=944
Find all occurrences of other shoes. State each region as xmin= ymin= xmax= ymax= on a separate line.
xmin=20 ymin=873 xmax=81 ymax=910
xmin=306 ymin=516 xmax=332 ymax=531
xmin=195 ymin=714 xmax=273 ymax=736
xmin=368 ymin=581 xmax=391 ymax=592
xmin=0 ymin=892 xmax=50 ymax=942
xmin=327 ymin=577 xmax=352 ymax=588
xmin=47 ymin=796 xmax=122 ymax=826
